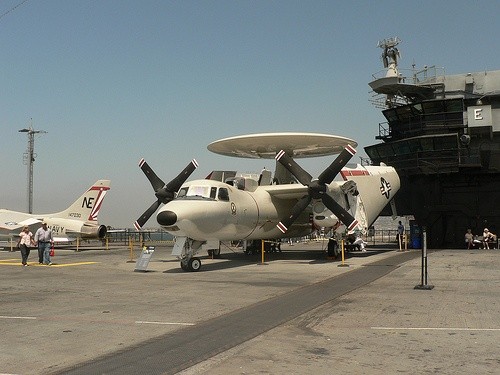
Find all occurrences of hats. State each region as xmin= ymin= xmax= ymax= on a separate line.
xmin=42 ymin=221 xmax=48 ymax=225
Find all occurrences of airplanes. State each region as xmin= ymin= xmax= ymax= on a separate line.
xmin=0 ymin=179 xmax=126 ymax=249
xmin=133 ymin=144 xmax=401 ymax=271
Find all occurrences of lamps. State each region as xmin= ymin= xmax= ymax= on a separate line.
xmin=476 ymin=95 xmax=487 ymax=105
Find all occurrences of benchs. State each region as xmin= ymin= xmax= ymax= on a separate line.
xmin=466 ymin=235 xmax=497 ymax=249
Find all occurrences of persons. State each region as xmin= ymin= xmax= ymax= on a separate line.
xmin=397 ymin=221 xmax=404 ymax=249
xmin=353 ymin=237 xmax=369 ymax=252
xmin=17 ymin=225 xmax=37 ymax=267
xmin=34 ymin=222 xmax=54 ymax=265
xmin=483 ymin=228 xmax=494 ymax=250
xmin=465 ymin=229 xmax=475 ymax=250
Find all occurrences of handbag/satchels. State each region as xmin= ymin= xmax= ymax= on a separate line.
xmin=50 ymin=243 xmax=55 ymax=256
